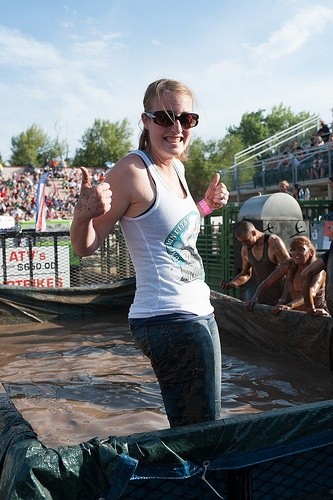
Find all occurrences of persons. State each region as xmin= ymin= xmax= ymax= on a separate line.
xmin=301 ymin=240 xmax=332 ymax=317
xmin=70 ymin=79 xmax=229 ymax=426
xmin=278 ymin=180 xmax=294 ymax=196
xmin=220 ymin=221 xmax=293 ymax=306
xmin=261 ymin=120 xmax=333 ymax=180
xmin=245 ymin=236 xmax=328 ymax=314
xmin=0 ymin=161 xmax=107 ymax=226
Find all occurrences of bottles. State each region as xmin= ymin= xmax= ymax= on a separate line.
xmin=305 ymin=188 xmax=310 ymax=200
xmin=299 ymin=188 xmax=304 ymax=200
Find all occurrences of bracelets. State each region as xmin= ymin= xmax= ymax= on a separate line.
xmin=199 ymin=199 xmax=213 ymax=215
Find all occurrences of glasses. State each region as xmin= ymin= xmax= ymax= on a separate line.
xmin=143 ymin=110 xmax=199 ymax=129
